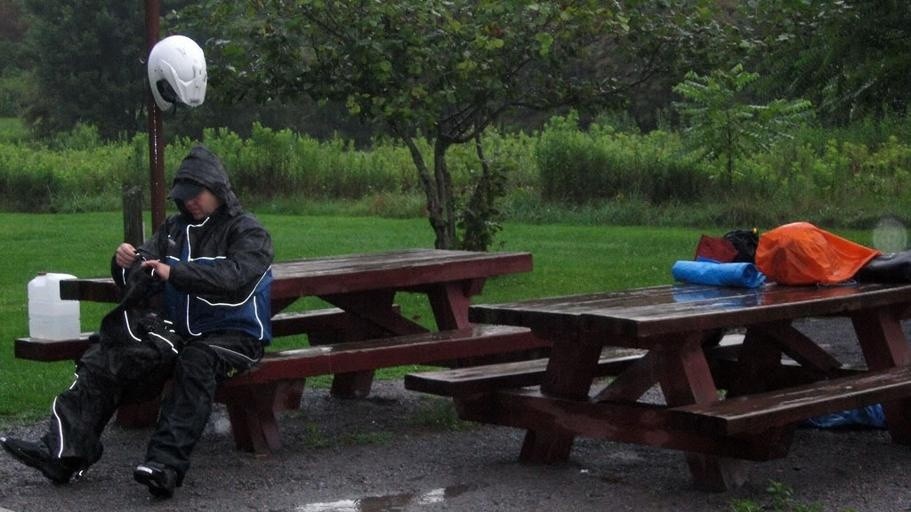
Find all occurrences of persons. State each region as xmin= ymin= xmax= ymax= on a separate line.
xmin=2 ymin=144 xmax=278 ymax=500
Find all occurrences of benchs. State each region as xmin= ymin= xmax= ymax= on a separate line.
xmin=220 ymin=324 xmax=558 ymax=389
xmin=666 ymin=362 xmax=911 ymax=438
xmin=13 ymin=305 xmax=402 ymax=363
xmin=406 ymin=332 xmax=747 ymax=400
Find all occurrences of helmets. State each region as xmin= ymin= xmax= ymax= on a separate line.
xmin=147 ymin=35 xmax=208 ymax=111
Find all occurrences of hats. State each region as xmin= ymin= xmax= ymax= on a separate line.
xmin=166 ymin=178 xmax=204 ymax=201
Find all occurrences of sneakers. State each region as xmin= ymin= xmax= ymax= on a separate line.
xmin=132 ymin=457 xmax=178 ymax=499
xmin=2 ymin=434 xmax=72 ymax=483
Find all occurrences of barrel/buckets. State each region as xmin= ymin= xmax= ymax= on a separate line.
xmin=28 ymin=272 xmax=80 ymax=340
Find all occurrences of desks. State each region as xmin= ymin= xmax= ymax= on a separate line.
xmin=60 ymin=247 xmax=534 ymax=457
xmin=469 ymin=279 xmax=908 ymax=494
